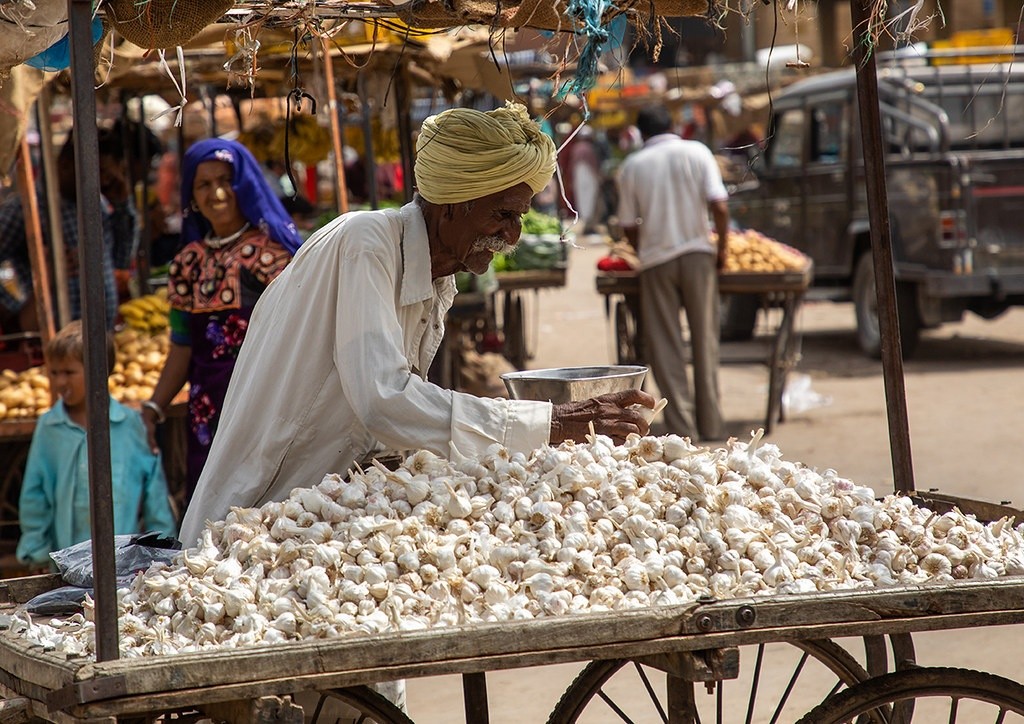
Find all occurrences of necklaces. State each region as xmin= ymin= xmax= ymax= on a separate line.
xmin=204 ymin=220 xmax=252 ymax=248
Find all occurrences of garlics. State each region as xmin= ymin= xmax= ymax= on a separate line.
xmin=51 ymin=399 xmax=1024 ymax=657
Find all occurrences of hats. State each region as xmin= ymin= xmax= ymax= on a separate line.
xmin=414 ymin=98 xmax=559 ymax=203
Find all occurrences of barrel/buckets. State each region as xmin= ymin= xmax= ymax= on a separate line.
xmin=499 ymin=366 xmax=649 ymax=405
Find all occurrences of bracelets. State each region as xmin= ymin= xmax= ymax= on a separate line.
xmin=139 ymin=400 xmax=165 ymax=426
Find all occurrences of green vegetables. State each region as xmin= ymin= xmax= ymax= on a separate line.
xmin=456 ymin=206 xmax=564 ymax=296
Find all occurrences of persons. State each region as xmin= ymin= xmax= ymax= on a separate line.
xmin=177 ymin=99 xmax=655 ymax=719
xmin=1 ymin=115 xmax=180 ymax=352
xmin=140 ymin=137 xmax=309 ymax=511
xmin=267 ymin=145 xmax=371 ymax=221
xmin=528 ymin=102 xmax=729 ymax=442
xmin=15 ymin=319 xmax=175 ymax=577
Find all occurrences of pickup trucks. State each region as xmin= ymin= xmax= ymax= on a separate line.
xmin=688 ymin=40 xmax=1023 ymax=360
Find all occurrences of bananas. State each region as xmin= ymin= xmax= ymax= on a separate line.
xmin=117 ymin=286 xmax=171 ymax=329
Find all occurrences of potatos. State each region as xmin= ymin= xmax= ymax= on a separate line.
xmin=710 ymin=229 xmax=788 ymax=272
xmin=0 ymin=329 xmax=169 ymax=417
